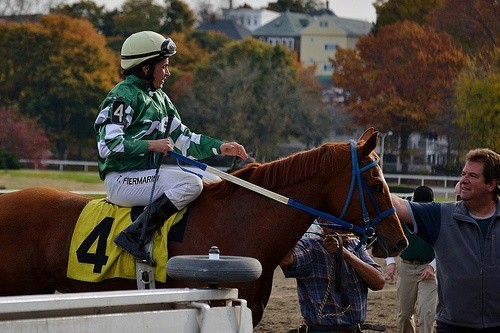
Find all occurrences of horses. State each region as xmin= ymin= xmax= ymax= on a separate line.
xmin=0 ymin=127 xmax=409 ymax=329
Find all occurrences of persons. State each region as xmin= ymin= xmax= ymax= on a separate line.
xmin=389 ymin=149 xmax=500 ymax=333
xmin=278 ymin=215 xmax=385 ymax=333
xmin=385 ymin=186 xmax=438 ymax=333
xmin=93 ymin=31 xmax=248 ymax=268
xmin=454 ymin=181 xmax=461 ymax=207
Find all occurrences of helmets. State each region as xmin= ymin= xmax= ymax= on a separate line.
xmin=121 ymin=31 xmax=176 ymax=70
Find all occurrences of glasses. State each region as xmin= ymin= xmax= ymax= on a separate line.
xmin=150 ymin=37 xmax=176 ymax=63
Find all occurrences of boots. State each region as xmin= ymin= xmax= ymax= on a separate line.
xmin=113 ymin=193 xmax=179 ymax=268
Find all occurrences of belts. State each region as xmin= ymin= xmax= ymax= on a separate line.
xmin=308 ymin=323 xmax=385 ymax=333
xmin=403 ymin=259 xmax=429 ymax=264
